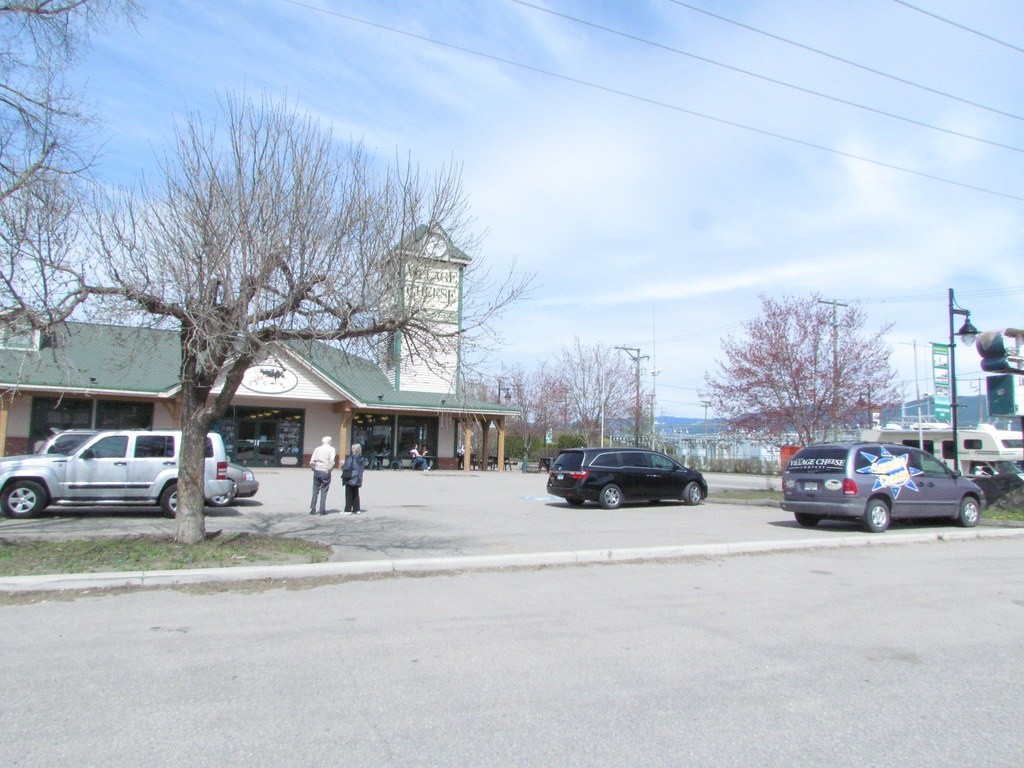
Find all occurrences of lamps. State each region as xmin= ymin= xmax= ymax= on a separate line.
xmin=377 ymin=395 xmax=383 ymax=400
xmin=91 ymin=377 xmax=96 ymax=382
xmin=442 ymin=400 xmax=447 ymax=404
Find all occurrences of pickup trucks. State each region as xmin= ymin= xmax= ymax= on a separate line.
xmin=0 ymin=427 xmax=260 ymax=520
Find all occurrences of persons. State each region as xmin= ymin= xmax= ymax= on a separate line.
xmin=340 ymin=443 xmax=365 ymax=514
xmin=309 ymin=436 xmax=336 ymax=515
xmin=410 ymin=444 xmax=433 ymax=472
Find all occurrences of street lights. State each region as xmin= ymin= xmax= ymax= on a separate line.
xmin=496 ymin=387 xmax=513 ymax=456
xmin=947 ymin=309 xmax=983 ymax=473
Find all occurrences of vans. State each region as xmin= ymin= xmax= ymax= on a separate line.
xmin=780 ymin=441 xmax=988 ymax=533
xmin=546 ymin=448 xmax=708 ymax=505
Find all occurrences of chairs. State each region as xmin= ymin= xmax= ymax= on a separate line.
xmin=470 ymin=454 xmax=477 ymax=469
xmin=489 ymin=456 xmax=511 ymax=471
xmin=391 ymin=451 xmax=403 ymax=470
xmin=364 ymin=450 xmax=390 ymax=470
xmin=409 ymin=452 xmax=424 ymax=470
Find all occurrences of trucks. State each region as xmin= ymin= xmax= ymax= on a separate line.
xmin=860 ymin=429 xmax=1024 ymax=478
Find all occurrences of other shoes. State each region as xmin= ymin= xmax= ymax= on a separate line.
xmin=356 ymin=510 xmax=362 ymax=514
xmin=310 ymin=508 xmax=316 ymax=514
xmin=423 ymin=466 xmax=432 ymax=471
xmin=339 ymin=508 xmax=352 ymax=515
xmin=320 ymin=510 xmax=326 ymax=514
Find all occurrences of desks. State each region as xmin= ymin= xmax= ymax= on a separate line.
xmin=425 ymin=456 xmax=437 ymax=467
xmin=480 ymin=459 xmax=493 ymax=468
xmin=375 ymin=455 xmax=388 ymax=469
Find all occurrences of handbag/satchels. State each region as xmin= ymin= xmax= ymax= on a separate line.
xmin=342 ymin=454 xmax=354 ymax=479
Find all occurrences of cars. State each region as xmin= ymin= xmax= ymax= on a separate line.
xmin=457 ymin=445 xmax=473 ymax=454
